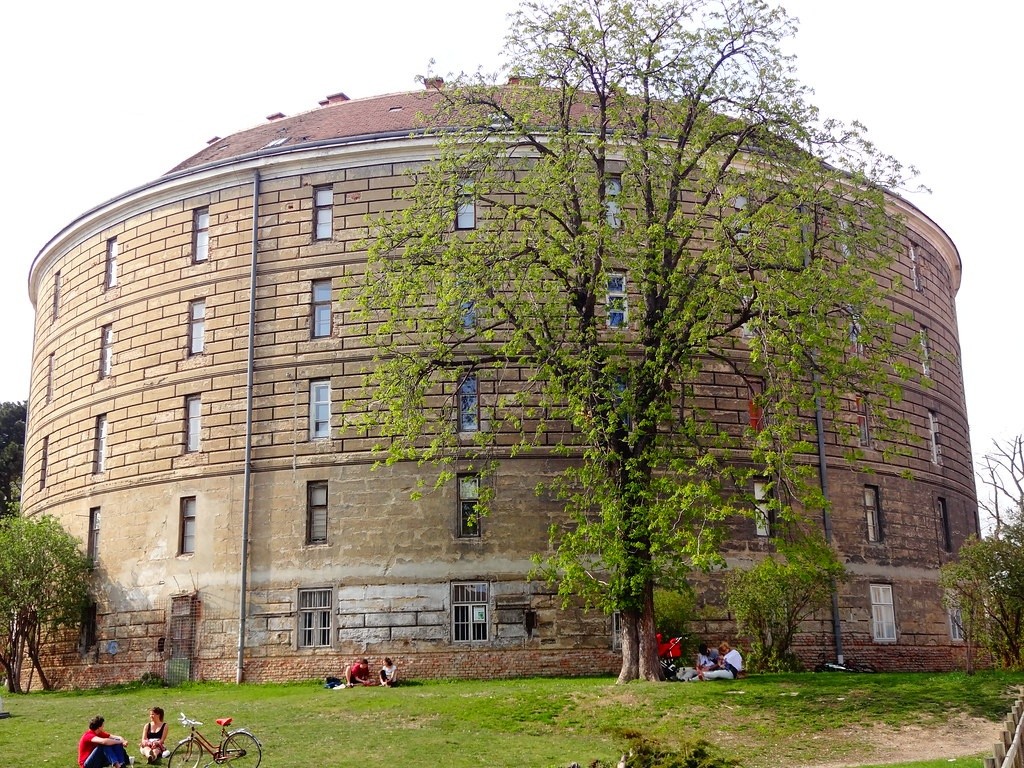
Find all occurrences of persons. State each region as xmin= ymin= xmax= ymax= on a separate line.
xmin=78 ymin=716 xmax=130 ymax=768
xmin=379 ymin=657 xmax=397 ymax=687
xmin=696 ymin=641 xmax=742 ymax=681
xmin=140 ymin=707 xmax=168 ymax=764
xmin=345 ymin=659 xmax=368 ymax=688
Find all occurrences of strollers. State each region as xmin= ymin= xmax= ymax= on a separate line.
xmin=653 ymin=626 xmax=690 ymax=683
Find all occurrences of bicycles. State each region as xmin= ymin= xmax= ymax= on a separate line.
xmin=167 ymin=712 xmax=263 ymax=768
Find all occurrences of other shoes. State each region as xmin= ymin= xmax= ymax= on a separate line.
xmin=348 ymin=685 xmax=352 ymax=688
xmin=698 ymin=674 xmax=705 ymax=681
xmin=697 ymin=667 xmax=703 ymax=674
xmin=153 ymin=752 xmax=162 ymax=765
xmin=147 ymin=755 xmax=153 ymax=764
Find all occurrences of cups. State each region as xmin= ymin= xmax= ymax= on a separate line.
xmin=129 ymin=757 xmax=135 ymax=764
xmin=162 ymin=750 xmax=170 ymax=758
